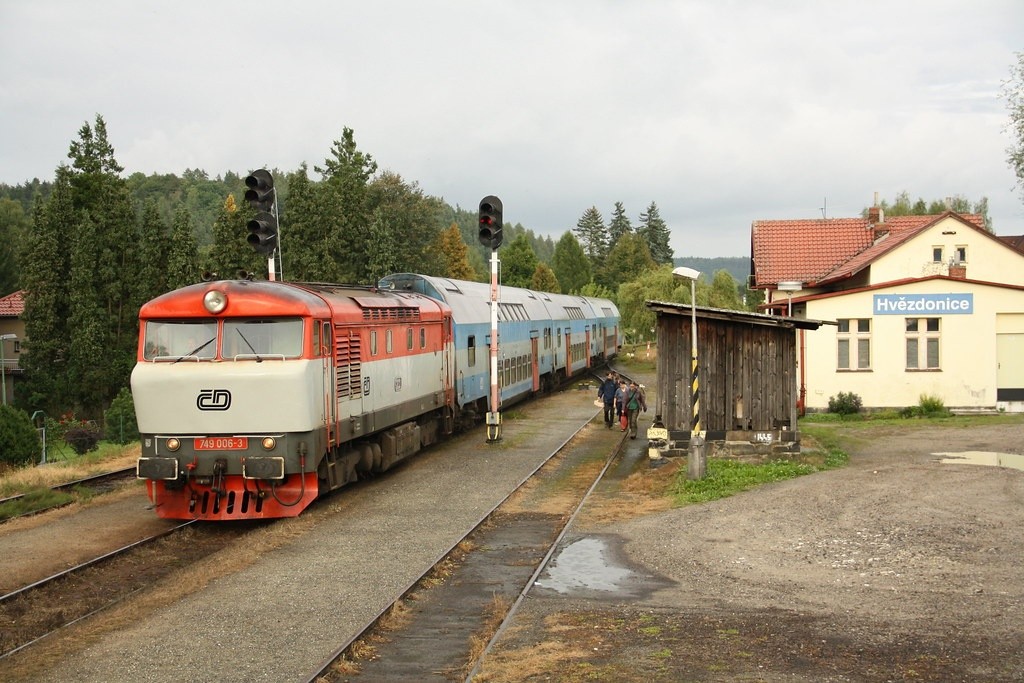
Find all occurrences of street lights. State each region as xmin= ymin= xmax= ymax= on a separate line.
xmin=777 ymin=281 xmax=802 ymax=316
xmin=672 ymin=265 xmax=708 ymax=479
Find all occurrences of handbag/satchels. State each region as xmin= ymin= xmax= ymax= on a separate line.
xmin=620 ymin=415 xmax=628 ymax=430
xmin=594 ymin=399 xmax=605 ymax=408
xmin=625 ymin=406 xmax=629 ymax=415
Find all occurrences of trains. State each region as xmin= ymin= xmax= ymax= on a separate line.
xmin=130 ymin=273 xmax=622 ymax=519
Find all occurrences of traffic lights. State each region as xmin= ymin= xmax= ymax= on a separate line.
xmin=245 ymin=168 xmax=277 ymax=253
xmin=479 ymin=195 xmax=503 ymax=249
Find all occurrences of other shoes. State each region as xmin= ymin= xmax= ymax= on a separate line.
xmin=606 ymin=421 xmax=609 ymax=426
xmin=630 ymin=432 xmax=636 ymax=439
xmin=609 ymin=424 xmax=613 ymax=429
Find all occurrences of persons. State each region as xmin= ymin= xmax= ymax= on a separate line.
xmin=622 ymin=381 xmax=648 ymax=440
xmin=598 ymin=371 xmax=618 ymax=430
xmin=613 ymin=380 xmax=630 ymax=432
xmin=613 ymin=373 xmax=622 ymax=394
